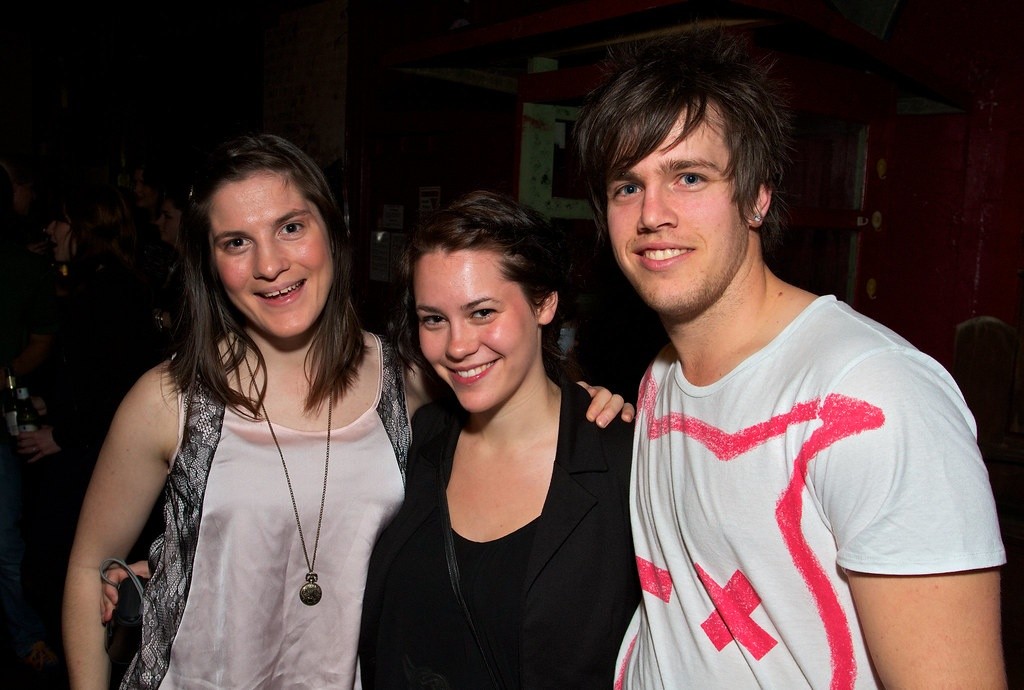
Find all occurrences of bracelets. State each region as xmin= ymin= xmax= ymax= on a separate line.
xmin=152 ymin=308 xmax=164 ymax=332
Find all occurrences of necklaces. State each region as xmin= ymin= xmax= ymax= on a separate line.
xmin=244 ymin=356 xmax=333 ymax=606
xmin=62 ymin=27 xmax=1008 ymax=690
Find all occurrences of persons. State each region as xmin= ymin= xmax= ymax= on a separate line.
xmin=0 ymin=164 xmax=190 ymax=671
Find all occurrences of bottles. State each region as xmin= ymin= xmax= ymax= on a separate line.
xmin=2 ymin=367 xmax=47 ymax=466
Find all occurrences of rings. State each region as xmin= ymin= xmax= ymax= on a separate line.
xmin=32 ymin=446 xmax=37 ymax=453
